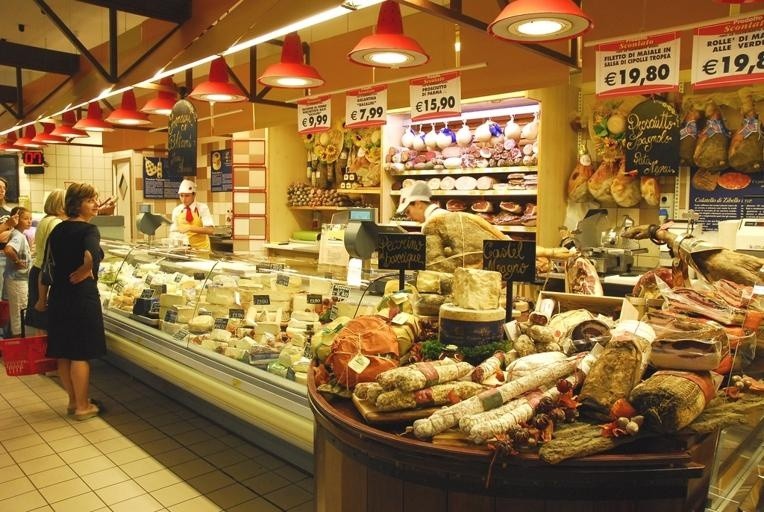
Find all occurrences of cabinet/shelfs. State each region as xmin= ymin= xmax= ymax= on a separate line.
xmin=705 ymin=259 xmax=762 ymax=510
xmin=92 ymin=231 xmax=454 ymax=480
xmin=381 ymin=166 xmax=541 ymax=236
xmin=288 ymin=188 xmax=380 ymax=212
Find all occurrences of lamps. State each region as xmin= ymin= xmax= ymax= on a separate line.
xmin=345 ymin=0 xmax=432 ymax=68
xmin=0 ymin=56 xmax=182 ymax=155
xmin=484 ymin=1 xmax=596 ymax=45
xmin=254 ymin=33 xmax=325 ymax=89
xmin=186 ymin=57 xmax=249 ymax=106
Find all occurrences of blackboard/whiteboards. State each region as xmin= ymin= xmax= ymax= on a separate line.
xmin=168 ymin=99 xmax=197 ymax=176
xmin=625 ymin=99 xmax=679 ymax=177
xmin=483 ymin=239 xmax=536 ymax=284
xmin=378 ymin=233 xmax=426 ymax=270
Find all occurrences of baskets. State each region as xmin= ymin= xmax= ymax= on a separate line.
xmin=0 ymin=307 xmax=57 ymax=377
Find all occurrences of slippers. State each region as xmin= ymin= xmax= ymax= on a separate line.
xmin=74 ymin=399 xmax=106 ymax=421
xmin=67 ymin=398 xmax=97 ymax=416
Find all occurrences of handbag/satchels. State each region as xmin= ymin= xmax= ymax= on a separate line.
xmin=41 ymin=263 xmax=57 ymax=285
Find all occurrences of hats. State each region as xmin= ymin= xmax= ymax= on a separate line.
xmin=395 ymin=179 xmax=431 ymax=213
xmin=178 ymin=179 xmax=197 ymax=194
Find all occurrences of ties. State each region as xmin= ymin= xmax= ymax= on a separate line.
xmin=186 ymin=206 xmax=193 ymax=225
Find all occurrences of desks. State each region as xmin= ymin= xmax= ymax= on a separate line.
xmin=299 ymin=357 xmax=716 ymax=510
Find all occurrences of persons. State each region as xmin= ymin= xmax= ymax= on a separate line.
xmin=394 ymin=180 xmax=451 ymax=230
xmin=0 ymin=177 xmax=68 ymax=380
xmin=33 ymin=182 xmax=106 ymax=421
xmin=167 ymin=179 xmax=215 ymax=258
